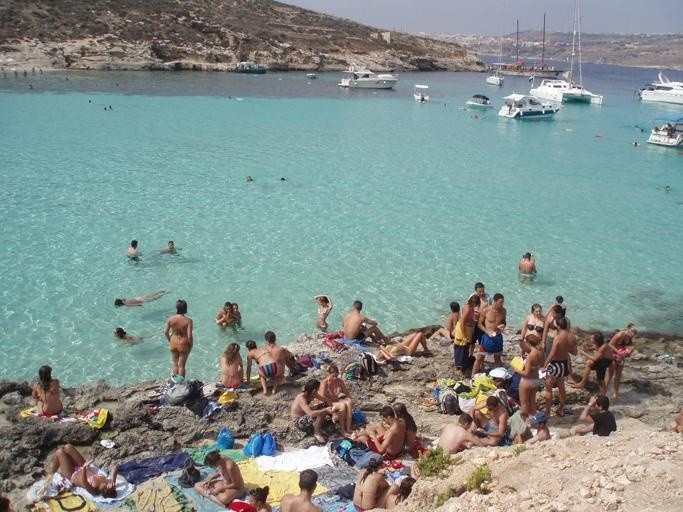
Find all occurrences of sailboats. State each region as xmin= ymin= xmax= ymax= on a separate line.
xmin=486 ymin=37 xmax=505 ymax=88
xmin=529 ymin=1 xmax=605 ymax=106
xmin=487 ymin=13 xmax=564 ymax=79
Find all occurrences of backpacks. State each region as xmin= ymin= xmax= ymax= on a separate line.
xmin=361 ymin=349 xmax=379 ymax=376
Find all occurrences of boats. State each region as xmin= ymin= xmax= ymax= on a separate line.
xmin=306 ymin=74 xmax=317 ymax=78
xmin=466 ymin=95 xmax=493 ymax=109
xmin=414 ymin=84 xmax=430 ymax=102
xmin=646 ymin=123 xmax=683 ymax=147
xmin=338 ymin=66 xmax=399 ymax=89
xmin=638 ymin=72 xmax=683 ymax=105
xmin=497 ymin=90 xmax=561 ymax=119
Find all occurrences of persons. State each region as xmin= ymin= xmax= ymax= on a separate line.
xmin=160 ymin=241 xmax=176 ymax=253
xmin=633 ymin=123 xmax=673 ymax=146
xmin=49 ymin=443 xmax=118 ymax=497
xmin=127 ymin=240 xmax=139 ymax=266
xmin=32 ymin=366 xmax=63 ymax=416
xmin=419 ymin=92 xmax=425 ymax=104
xmin=166 ymin=295 xmax=485 ymax=512
xmin=246 ymin=176 xmax=255 ymax=183
xmin=448 ymin=281 xmax=636 ymax=446
xmin=112 ymin=327 xmax=164 ymax=344
xmin=518 ymin=252 xmax=537 ymax=281
xmin=504 ymin=101 xmax=512 ymax=114
xmin=115 ymin=289 xmax=169 ymax=306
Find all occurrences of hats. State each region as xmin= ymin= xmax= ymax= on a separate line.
xmin=367 ymin=451 xmax=385 ymax=466
xmin=528 ymin=411 xmax=548 ymax=427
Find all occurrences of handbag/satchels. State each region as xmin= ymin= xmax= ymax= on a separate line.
xmin=217 ymin=427 xmax=234 ymax=450
xmin=178 ymin=457 xmax=201 ymax=488
xmin=243 ymin=431 xmax=277 ymax=457
